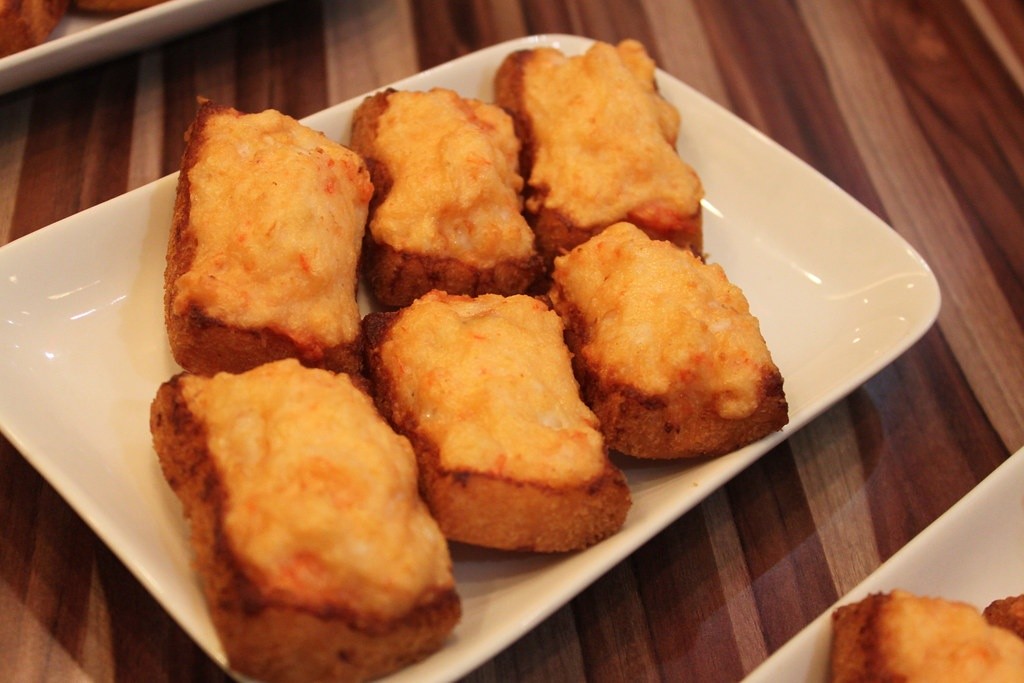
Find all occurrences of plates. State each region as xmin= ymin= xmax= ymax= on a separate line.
xmin=1 ymin=30 xmax=945 ymax=683
xmin=0 ymin=1 xmax=261 ymax=97
xmin=734 ymin=441 xmax=1024 ymax=683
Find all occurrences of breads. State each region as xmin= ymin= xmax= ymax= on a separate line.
xmin=544 ymin=217 xmax=793 ymax=455
xmin=163 ymin=98 xmax=375 ymax=373
xmin=819 ymin=584 xmax=1024 ymax=682
xmin=143 ymin=355 xmax=463 ymax=677
xmin=494 ymin=33 xmax=708 ymax=274
xmin=349 ymin=286 xmax=631 ymax=559
xmin=341 ymin=88 xmax=547 ymax=314
